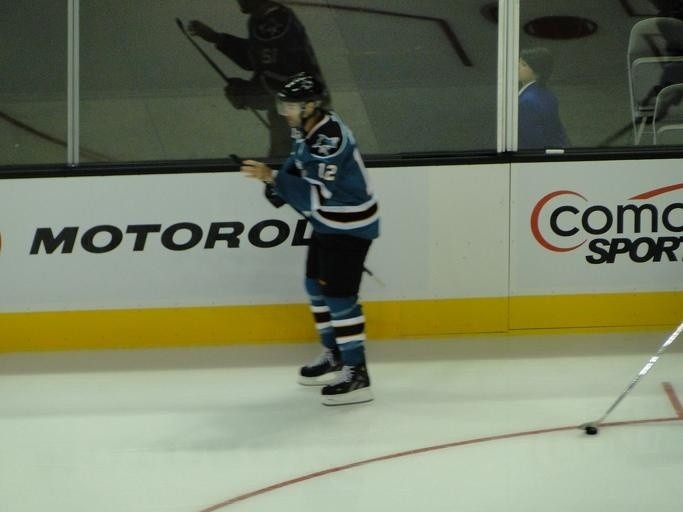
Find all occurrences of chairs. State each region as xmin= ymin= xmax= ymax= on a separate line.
xmin=625 ymin=18 xmax=682 ymax=147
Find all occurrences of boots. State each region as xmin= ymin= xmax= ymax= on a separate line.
xmin=319 ymin=359 xmax=371 ymax=396
xmin=298 ymin=348 xmax=344 ymax=378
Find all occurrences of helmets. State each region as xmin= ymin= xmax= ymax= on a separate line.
xmin=275 ymin=75 xmax=327 ymax=103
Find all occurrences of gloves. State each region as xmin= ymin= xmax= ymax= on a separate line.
xmin=265 ymin=180 xmax=286 ymax=209
xmin=224 ymin=76 xmax=253 ymax=111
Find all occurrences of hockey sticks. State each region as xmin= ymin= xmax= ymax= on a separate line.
xmin=229 ymin=154 xmax=389 ymax=291
xmin=582 ymin=322 xmax=682 ymax=429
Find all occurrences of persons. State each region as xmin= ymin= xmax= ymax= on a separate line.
xmin=237 ymin=74 xmax=381 ymax=396
xmin=516 ymin=48 xmax=570 ymax=150
xmin=186 ymin=0 xmax=332 ymax=159
